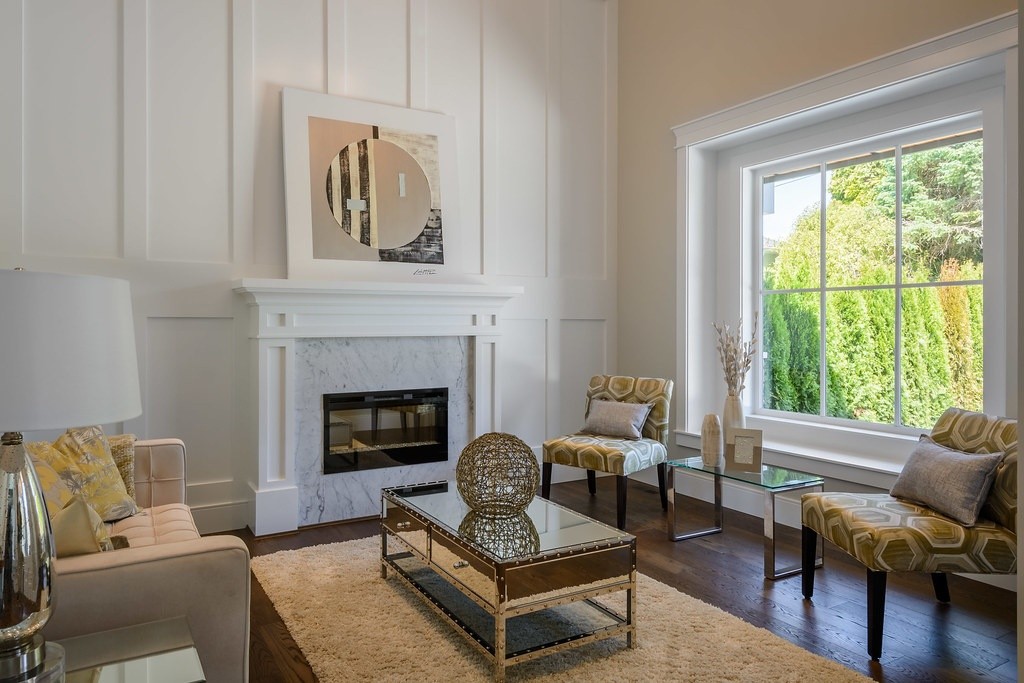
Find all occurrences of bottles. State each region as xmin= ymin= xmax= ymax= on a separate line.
xmin=701 ymin=414 xmax=722 ymax=467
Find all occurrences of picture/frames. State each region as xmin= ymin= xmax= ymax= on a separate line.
xmin=281 ymin=84 xmax=466 ymax=285
xmin=725 ymin=428 xmax=762 ymax=473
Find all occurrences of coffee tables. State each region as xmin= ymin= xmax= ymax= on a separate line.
xmin=380 ymin=480 xmax=637 ymax=681
xmin=329 ymin=413 xmax=353 ymax=449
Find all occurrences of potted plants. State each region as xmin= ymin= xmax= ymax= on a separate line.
xmin=711 ymin=311 xmax=758 ymax=396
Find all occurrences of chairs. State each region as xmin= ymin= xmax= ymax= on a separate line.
xmin=541 ymin=375 xmax=674 ymax=530
xmin=371 ymin=405 xmax=435 ymax=443
xmin=801 ymin=406 xmax=1017 ymax=659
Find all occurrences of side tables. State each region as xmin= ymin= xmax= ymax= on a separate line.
xmin=50 ymin=615 xmax=206 ymax=683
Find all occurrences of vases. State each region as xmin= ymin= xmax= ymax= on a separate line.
xmin=722 ymin=395 xmax=745 ymax=451
xmin=701 ymin=413 xmax=723 ymax=468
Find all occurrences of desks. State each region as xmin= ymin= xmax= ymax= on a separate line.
xmin=666 ymin=456 xmax=824 ymax=580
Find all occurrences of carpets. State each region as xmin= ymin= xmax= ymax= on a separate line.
xmin=250 ymin=530 xmax=879 ymax=683
xmin=330 ymin=441 xmax=438 ymax=456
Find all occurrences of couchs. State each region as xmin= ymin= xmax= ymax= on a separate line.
xmin=38 ymin=438 xmax=250 ymax=683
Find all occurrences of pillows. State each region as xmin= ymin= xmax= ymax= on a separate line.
xmin=890 ymin=434 xmax=1005 ymax=528
xmin=51 ymin=493 xmax=114 ymax=558
xmin=24 ymin=424 xmax=141 ymax=521
xmin=30 ymin=452 xmax=72 ymax=518
xmin=26 ymin=448 xmax=74 ymax=521
xmin=578 ymin=398 xmax=656 ymax=441
xmin=107 ymin=434 xmax=137 ymax=507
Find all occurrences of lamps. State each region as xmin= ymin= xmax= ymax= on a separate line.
xmin=0 ymin=268 xmax=142 ymax=683
